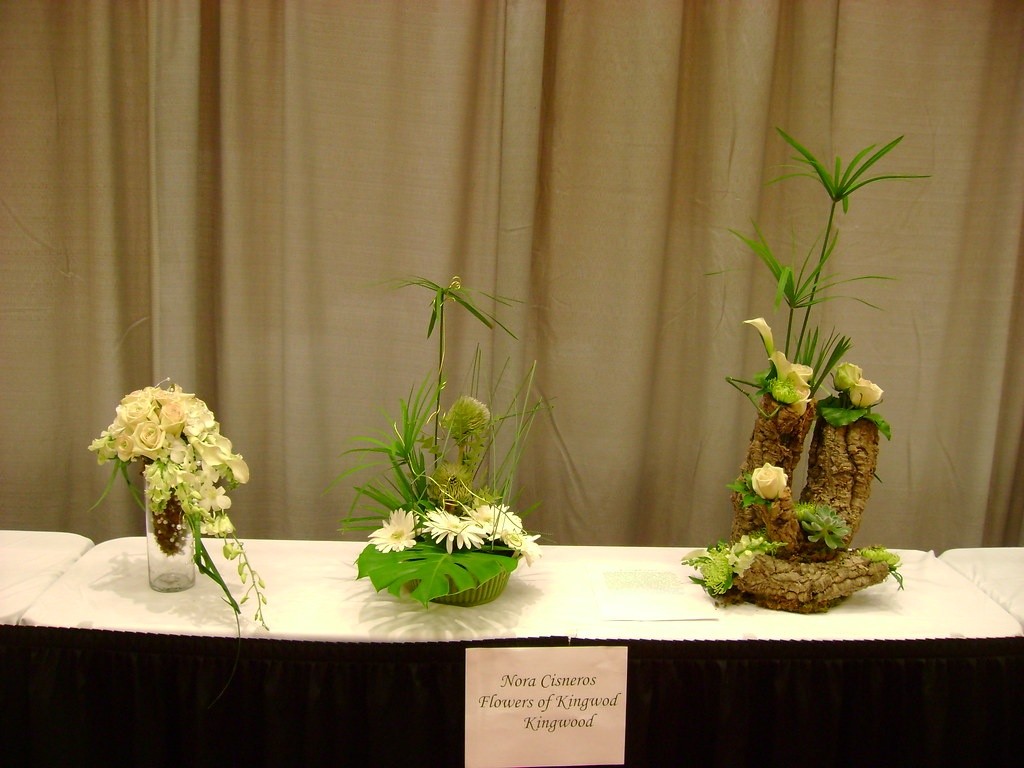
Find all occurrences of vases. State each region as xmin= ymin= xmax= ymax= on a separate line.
xmin=404 ymin=571 xmax=510 ymax=608
xmin=144 ymin=473 xmax=196 ymax=593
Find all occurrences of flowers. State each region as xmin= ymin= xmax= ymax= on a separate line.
xmin=684 ymin=120 xmax=934 ymax=597
xmin=331 ymin=255 xmax=549 ymax=594
xmin=80 ymin=377 xmax=270 ymax=632
